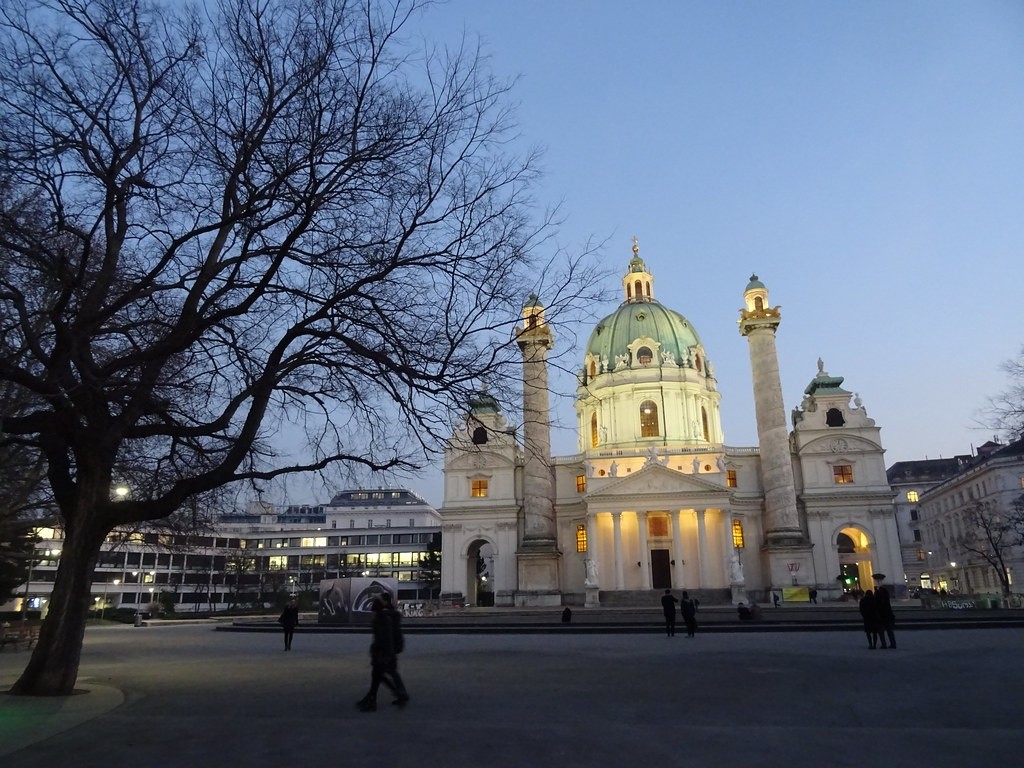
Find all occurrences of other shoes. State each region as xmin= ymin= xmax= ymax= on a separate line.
xmin=391 ymin=693 xmax=409 ymax=708
xmin=667 ymin=633 xmax=674 ymax=636
xmin=356 ymin=694 xmax=377 ymax=712
xmin=685 ymin=634 xmax=694 ymax=638
xmin=284 ymin=646 xmax=290 ymax=651
xmin=881 ymin=643 xmax=895 ymax=650
xmin=868 ymin=644 xmax=876 ymax=648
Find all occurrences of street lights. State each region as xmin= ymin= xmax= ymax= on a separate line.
xmin=132 ymin=567 xmax=156 ymax=627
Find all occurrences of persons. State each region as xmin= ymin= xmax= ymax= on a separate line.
xmin=661 ymin=589 xmax=701 ymax=638
xmin=808 ymin=587 xmax=817 ymax=604
xmin=692 ymin=456 xmax=704 ymax=473
xmin=773 ymin=591 xmax=781 ymax=609
xmin=354 ymin=592 xmax=411 ymax=713
xmin=852 ymin=585 xmax=897 ymax=650
xmin=281 ymin=598 xmax=300 ymax=652
xmin=737 ymin=600 xmax=762 ymax=620
xmin=715 ymin=454 xmax=727 ymax=473
xmin=609 ymin=460 xmax=620 ymax=477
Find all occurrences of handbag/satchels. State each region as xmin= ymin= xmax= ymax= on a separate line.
xmin=278 ymin=610 xmax=285 ymax=624
